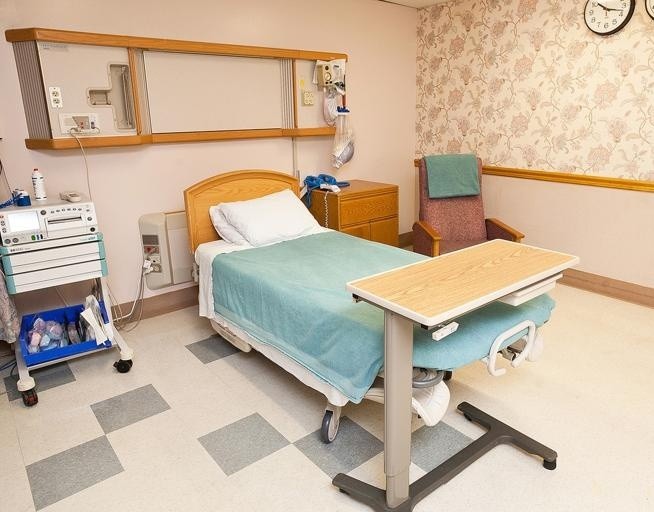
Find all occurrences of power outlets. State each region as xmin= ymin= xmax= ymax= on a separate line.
xmin=48 ymin=86 xmax=63 ymax=109
xmin=143 ymin=245 xmax=158 ymax=255
xmin=144 ymin=264 xmax=161 ymax=273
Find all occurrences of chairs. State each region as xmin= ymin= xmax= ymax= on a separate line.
xmin=412 ymin=155 xmax=525 ymax=257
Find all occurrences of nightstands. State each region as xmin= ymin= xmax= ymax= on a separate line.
xmin=308 ymin=178 xmax=399 ymax=247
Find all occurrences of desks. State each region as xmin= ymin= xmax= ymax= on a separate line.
xmin=329 ymin=236 xmax=579 ymax=511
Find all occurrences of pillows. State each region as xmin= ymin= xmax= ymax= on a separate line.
xmin=217 ymin=188 xmax=319 ymax=245
xmin=207 ymin=205 xmax=245 ymax=250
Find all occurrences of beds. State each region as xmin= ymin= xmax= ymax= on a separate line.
xmin=183 ymin=170 xmax=557 ymax=441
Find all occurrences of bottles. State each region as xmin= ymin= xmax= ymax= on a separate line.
xmin=16 ymin=190 xmax=31 ymax=206
xmin=335 ymin=105 xmax=351 ymax=146
xmin=31 ymin=168 xmax=48 ymax=201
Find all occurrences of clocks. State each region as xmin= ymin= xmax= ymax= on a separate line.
xmin=583 ymin=0 xmax=635 ymax=36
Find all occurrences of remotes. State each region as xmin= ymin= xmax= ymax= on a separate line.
xmin=67 ymin=193 xmax=81 ymax=202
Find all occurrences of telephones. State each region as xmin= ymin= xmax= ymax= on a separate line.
xmin=304 ymin=174 xmax=336 ymax=189
